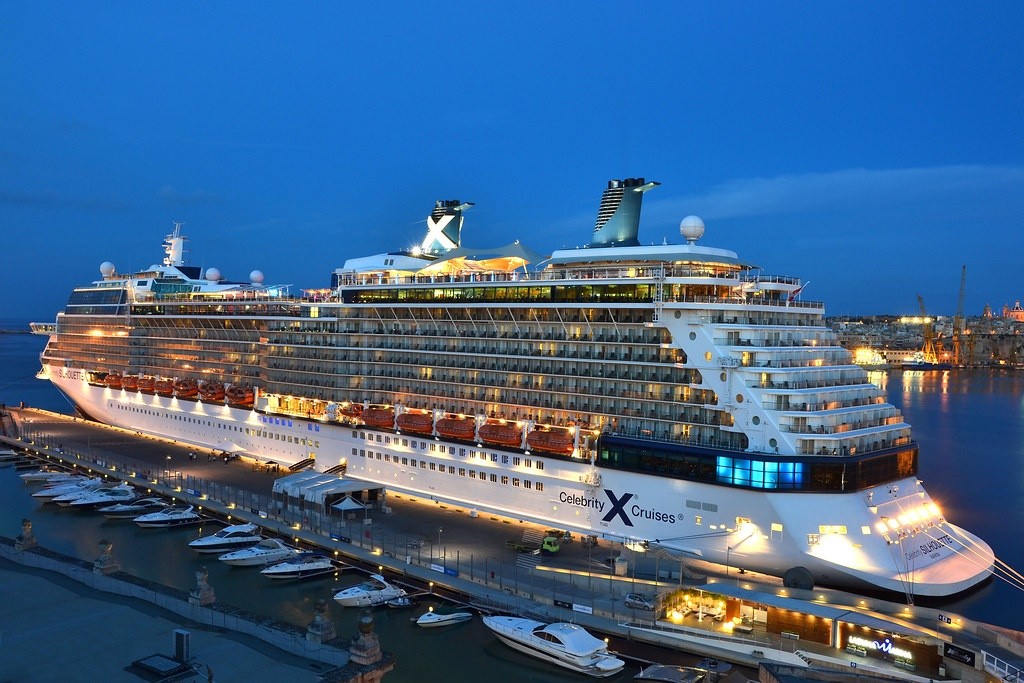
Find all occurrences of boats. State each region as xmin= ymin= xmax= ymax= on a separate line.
xmin=259 ymin=554 xmax=335 ymax=579
xmin=218 ymin=539 xmax=298 ymax=565
xmin=0 ymin=449 xmax=18 ymax=458
xmin=385 ymin=599 xmax=416 ymax=608
xmin=70 ymin=484 xmax=141 ymax=509
xmin=477 ymin=610 xmax=625 ymax=679
xmin=45 ymin=476 xmax=89 ymax=485
xmin=19 ymin=469 xmax=70 ymax=481
xmin=32 ymin=480 xmax=103 ymax=502
xmin=416 ymin=605 xmax=472 ymax=627
xmin=132 ymin=504 xmax=203 ymax=528
xmin=189 ymin=523 xmax=262 ymax=553
xmin=332 ymin=574 xmax=408 ymax=607
xmin=89 ymin=364 xmax=574 ymax=456
xmin=98 ymin=498 xmax=170 ymax=519
xmin=51 ymin=486 xmax=112 ymax=503
xmin=634 ymin=658 xmax=761 ymax=683
xmin=902 ymin=362 xmax=953 ymax=371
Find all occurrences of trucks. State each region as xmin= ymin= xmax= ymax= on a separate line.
xmin=505 ymin=536 xmax=559 ymax=556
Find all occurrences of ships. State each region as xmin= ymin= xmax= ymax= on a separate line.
xmin=30 ymin=176 xmax=995 ymax=605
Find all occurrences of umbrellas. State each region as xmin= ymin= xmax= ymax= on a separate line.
xmin=265 ymin=461 xmax=278 ymax=464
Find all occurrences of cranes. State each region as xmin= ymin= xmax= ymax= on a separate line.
xmin=916 ymin=264 xmax=987 ymax=365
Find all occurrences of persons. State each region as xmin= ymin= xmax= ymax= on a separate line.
xmin=193 ymin=452 xmax=196 ymax=460
xmin=208 ymin=449 xmax=235 ymax=464
xmin=277 ymin=464 xmax=279 ymax=472
xmin=189 ymin=452 xmax=193 ymax=460
xmin=267 ymin=466 xmax=269 ymax=473
xmin=741 ymin=614 xmax=748 ymax=623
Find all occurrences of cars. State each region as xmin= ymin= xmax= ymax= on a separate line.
xmin=625 ymin=592 xmax=655 ymax=611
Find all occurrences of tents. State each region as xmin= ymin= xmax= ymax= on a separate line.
xmin=330 ymin=496 xmax=367 ymax=522
xmin=214 ymin=442 xmax=246 ymax=452
